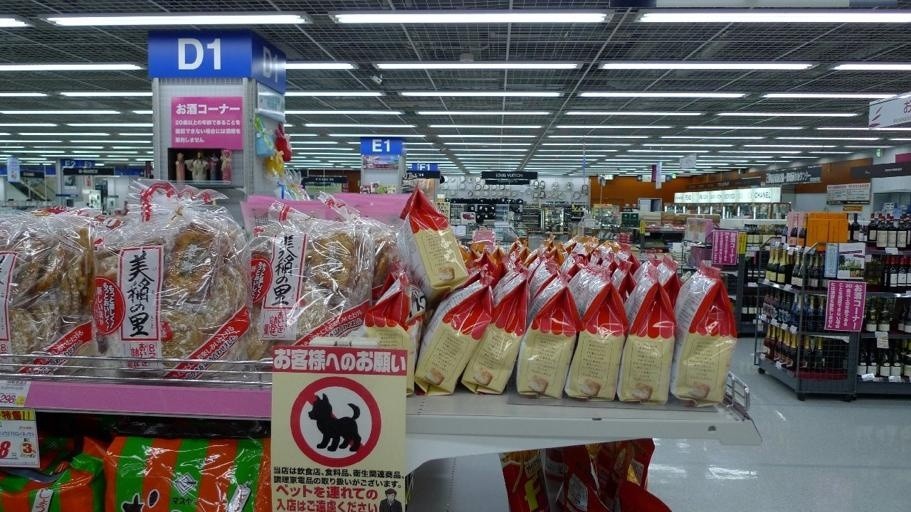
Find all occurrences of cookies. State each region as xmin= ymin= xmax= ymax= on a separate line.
xmin=0 ymin=220 xmax=396 ymax=378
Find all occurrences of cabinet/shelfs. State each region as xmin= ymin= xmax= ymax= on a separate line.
xmin=570 ymin=201 xmax=786 ymax=337
xmin=752 ymin=206 xmax=909 ymax=402
xmin=451 ymin=197 xmax=566 ymax=238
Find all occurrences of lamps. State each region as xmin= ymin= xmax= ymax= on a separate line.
xmin=0 ymin=10 xmax=911 ymax=173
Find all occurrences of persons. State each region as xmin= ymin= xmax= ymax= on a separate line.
xmin=175 ymin=150 xmax=232 ymax=180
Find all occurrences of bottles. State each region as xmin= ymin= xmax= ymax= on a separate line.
xmin=745 ymin=210 xmax=910 ymax=379
xmin=175 ymin=150 xmax=228 ymax=180
